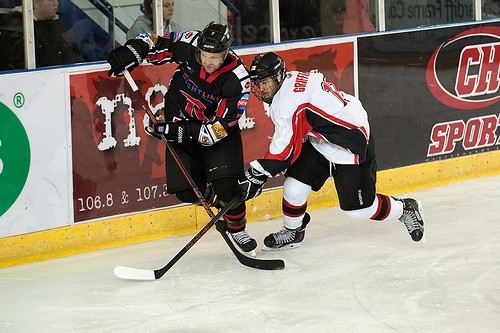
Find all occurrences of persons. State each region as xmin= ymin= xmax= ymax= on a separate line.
xmin=0 ymin=0 xmax=84 ymax=71
xmin=107 ymin=20 xmax=258 ymax=252
xmin=234 ymin=52 xmax=425 ymax=250
xmin=126 ymin=0 xmax=182 ymax=39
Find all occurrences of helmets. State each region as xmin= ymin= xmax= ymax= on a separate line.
xmin=249 ymin=52 xmax=286 ymax=104
xmin=197 ymin=21 xmax=233 ymax=54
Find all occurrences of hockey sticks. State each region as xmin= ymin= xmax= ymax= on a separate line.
xmin=114 ymin=192 xmax=243 ymax=280
xmin=123 ymin=70 xmax=285 ymax=271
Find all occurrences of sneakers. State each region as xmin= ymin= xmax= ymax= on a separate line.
xmin=230 ymin=231 xmax=258 ymax=259
xmin=390 ymin=195 xmax=426 ymax=243
xmin=261 ymin=211 xmax=311 ymax=250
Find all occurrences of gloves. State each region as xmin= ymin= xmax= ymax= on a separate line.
xmin=234 ymin=166 xmax=269 ymax=201
xmin=105 ymin=38 xmax=150 ymax=78
xmin=144 ymin=120 xmax=191 ymax=145
